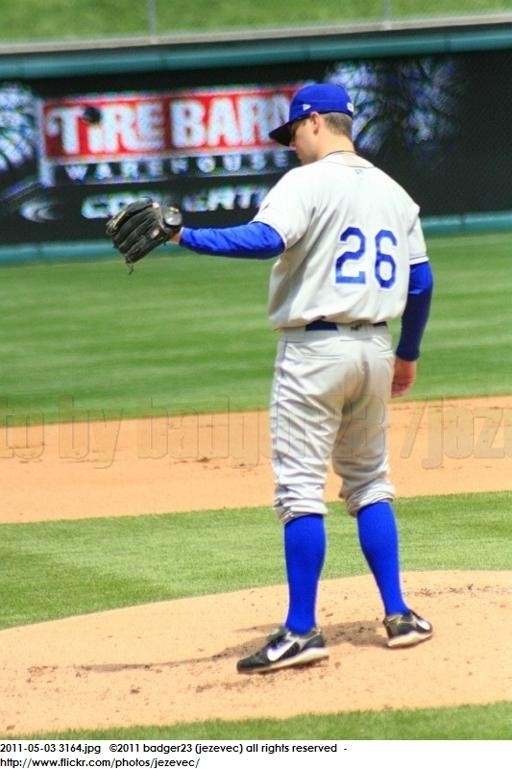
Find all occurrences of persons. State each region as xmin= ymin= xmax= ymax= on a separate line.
xmin=104 ymin=82 xmax=434 ymax=674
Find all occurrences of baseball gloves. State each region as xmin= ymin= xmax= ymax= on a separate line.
xmin=105 ymin=196 xmax=182 ymax=263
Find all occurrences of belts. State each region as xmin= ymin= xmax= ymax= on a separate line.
xmin=306 ymin=319 xmax=387 ymax=330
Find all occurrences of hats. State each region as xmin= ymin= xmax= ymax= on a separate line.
xmin=268 ymin=83 xmax=354 ymax=147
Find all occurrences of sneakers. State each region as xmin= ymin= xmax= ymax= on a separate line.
xmin=383 ymin=608 xmax=433 ymax=649
xmin=236 ymin=625 xmax=329 ymax=674
